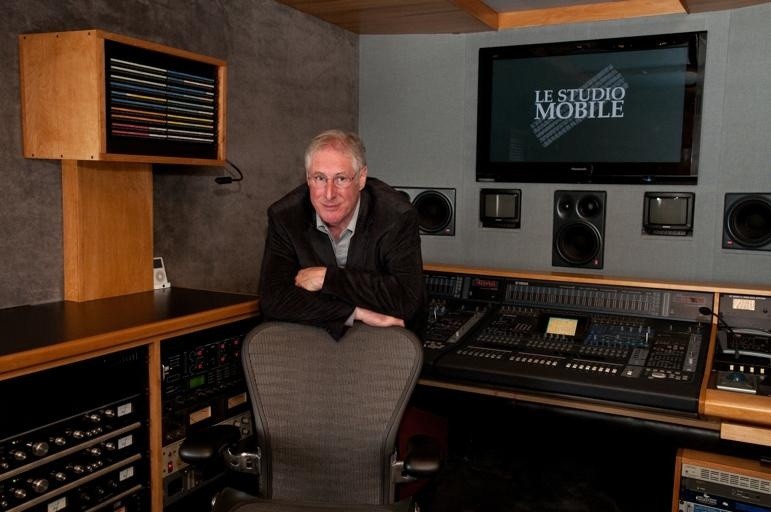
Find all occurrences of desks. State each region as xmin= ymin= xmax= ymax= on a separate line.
xmin=417 ymin=262 xmax=771 ymax=512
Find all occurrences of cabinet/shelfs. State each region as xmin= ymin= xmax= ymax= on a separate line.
xmin=18 ymin=27 xmax=228 ymax=168
xmin=0 ymin=281 xmax=261 ymax=511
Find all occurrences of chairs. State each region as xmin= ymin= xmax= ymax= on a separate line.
xmin=179 ymin=321 xmax=426 ymax=512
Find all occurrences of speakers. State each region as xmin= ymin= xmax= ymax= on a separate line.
xmin=394 ymin=187 xmax=455 ymax=236
xmin=721 ymin=192 xmax=770 ymax=250
xmin=551 ymin=189 xmax=608 ymax=270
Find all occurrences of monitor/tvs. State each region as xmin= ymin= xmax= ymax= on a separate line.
xmin=478 ymin=189 xmax=520 ymax=228
xmin=475 ymin=30 xmax=710 ymax=184
xmin=644 ymin=193 xmax=694 ymax=235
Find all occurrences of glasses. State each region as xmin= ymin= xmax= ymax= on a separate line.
xmin=307 ymin=166 xmax=362 ymax=188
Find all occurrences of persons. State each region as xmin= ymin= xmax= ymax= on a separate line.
xmin=258 ymin=129 xmax=429 ymax=350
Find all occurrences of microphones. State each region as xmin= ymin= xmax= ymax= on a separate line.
xmin=700 ymin=303 xmax=750 ymax=382
xmin=212 ymin=161 xmax=245 ymax=184
